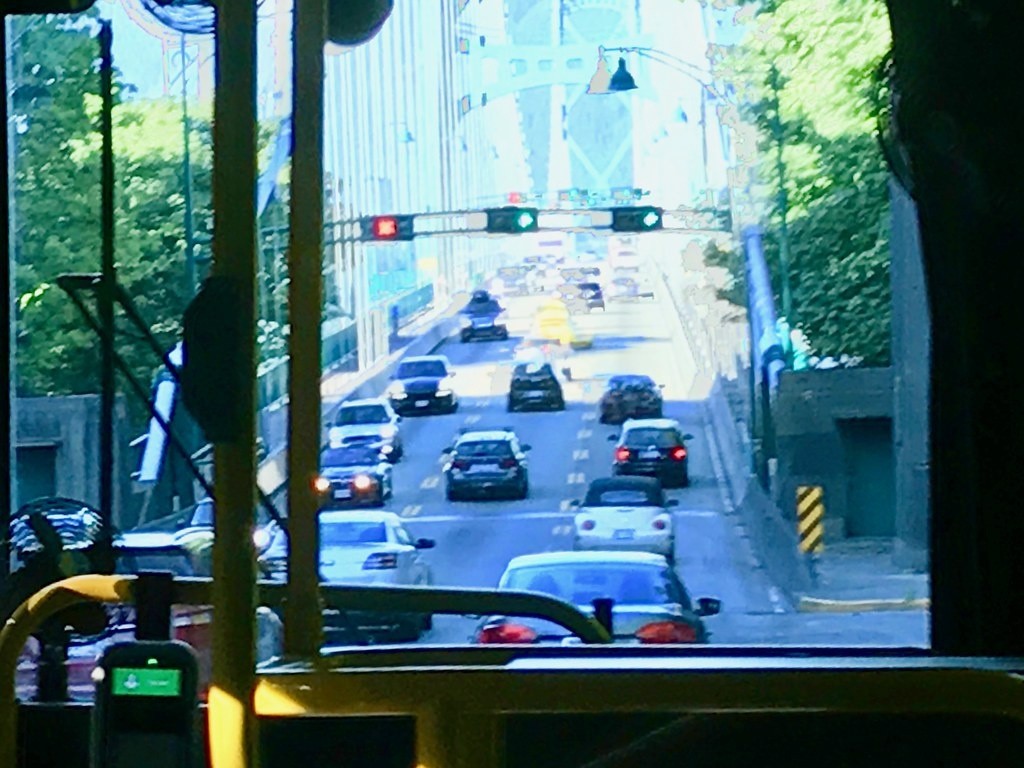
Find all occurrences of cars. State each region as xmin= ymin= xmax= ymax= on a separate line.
xmin=324 ymin=399 xmax=403 ymax=463
xmin=507 ymin=364 xmax=565 ymax=412
xmin=176 ymin=497 xmax=212 ymax=531
xmin=316 ymin=446 xmax=393 ymax=509
xmin=456 ymin=300 xmax=509 ymax=342
xmin=599 ymin=374 xmax=665 ymax=423
xmin=462 ymin=549 xmax=722 ymax=645
xmin=318 ymin=510 xmax=436 ymax=588
xmin=388 ymin=355 xmax=458 ymax=413
xmin=571 ymin=476 xmax=680 ymax=562
xmin=499 ymin=249 xmax=655 ymax=312
xmin=441 ymin=430 xmax=532 ymax=500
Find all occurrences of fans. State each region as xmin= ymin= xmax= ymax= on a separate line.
xmin=0 ymin=495 xmax=136 ymax=701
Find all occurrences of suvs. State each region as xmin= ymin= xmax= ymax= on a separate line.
xmin=607 ymin=418 xmax=695 ymax=488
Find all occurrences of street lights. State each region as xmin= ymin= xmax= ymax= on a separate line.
xmin=587 ymin=44 xmax=730 ymax=164
